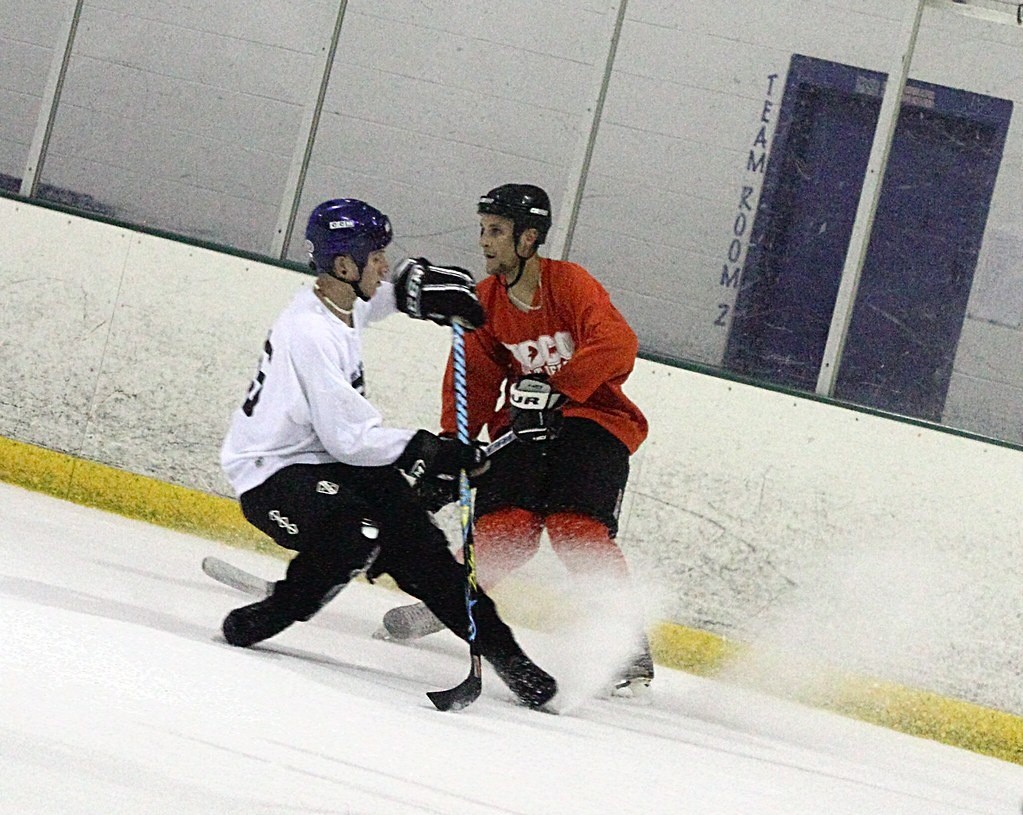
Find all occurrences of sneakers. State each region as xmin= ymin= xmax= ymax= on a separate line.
xmin=224 ymin=596 xmax=299 ymax=648
xmin=373 ymin=601 xmax=446 ymax=642
xmin=489 ymin=651 xmax=560 ymax=714
xmin=597 ymin=635 xmax=655 ymax=702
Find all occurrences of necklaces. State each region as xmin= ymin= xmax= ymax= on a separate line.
xmin=313 ymin=278 xmax=355 ymax=314
xmin=505 ymin=264 xmax=544 ymax=311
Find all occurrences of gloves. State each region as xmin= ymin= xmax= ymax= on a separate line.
xmin=391 ymin=257 xmax=484 ymax=331
xmin=507 ymin=371 xmax=570 ymax=443
xmin=417 ymin=436 xmax=495 ymax=511
xmin=393 ymin=430 xmax=485 ymax=493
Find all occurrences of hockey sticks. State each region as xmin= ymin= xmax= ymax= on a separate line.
xmin=204 ymin=425 xmax=519 ymax=623
xmin=427 ymin=316 xmax=482 ymax=710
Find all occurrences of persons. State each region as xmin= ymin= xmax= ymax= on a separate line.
xmin=386 ymin=183 xmax=656 ymax=703
xmin=217 ymin=200 xmax=559 ymax=710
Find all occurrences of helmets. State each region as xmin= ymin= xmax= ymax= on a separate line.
xmin=304 ymin=200 xmax=393 ymax=273
xmin=477 ymin=185 xmax=551 ymax=246
